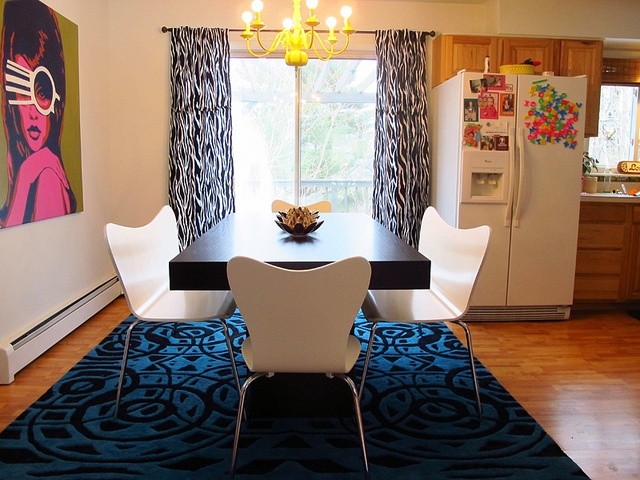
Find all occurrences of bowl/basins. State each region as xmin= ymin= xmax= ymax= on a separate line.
xmin=583 ymin=176 xmax=597 ymax=194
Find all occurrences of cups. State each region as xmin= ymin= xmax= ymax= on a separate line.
xmin=602 ymin=169 xmax=612 ymax=193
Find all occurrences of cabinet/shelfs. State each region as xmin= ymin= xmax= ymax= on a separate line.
xmin=431 ymin=33 xmax=601 ymax=139
xmin=570 ymin=199 xmax=640 ymax=310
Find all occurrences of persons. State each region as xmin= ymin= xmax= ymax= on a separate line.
xmin=480 ymin=97 xmax=497 ymax=118
xmin=502 ymin=96 xmax=513 ymax=113
xmin=0 ymin=0 xmax=77 ymax=228
xmin=465 ymin=132 xmax=477 ymax=145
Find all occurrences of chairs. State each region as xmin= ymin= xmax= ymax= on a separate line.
xmin=221 ymin=257 xmax=375 ymax=474
xmin=270 ymin=199 xmax=332 ymax=213
xmin=360 ymin=208 xmax=491 ymax=419
xmin=104 ymin=203 xmax=245 ymax=418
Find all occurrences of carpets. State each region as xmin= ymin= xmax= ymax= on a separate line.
xmin=1 ymin=311 xmax=588 ymax=480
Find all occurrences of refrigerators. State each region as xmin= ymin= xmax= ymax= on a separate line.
xmin=423 ymin=69 xmax=589 ymax=323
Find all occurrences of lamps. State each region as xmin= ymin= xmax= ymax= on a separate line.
xmin=239 ymin=0 xmax=358 ymax=67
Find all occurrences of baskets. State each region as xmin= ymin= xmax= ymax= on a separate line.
xmin=500 ymin=64 xmax=533 ymax=74
